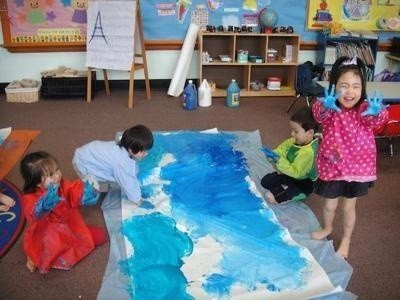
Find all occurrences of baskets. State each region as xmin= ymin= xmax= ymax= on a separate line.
xmin=5 ymin=80 xmax=43 ymax=104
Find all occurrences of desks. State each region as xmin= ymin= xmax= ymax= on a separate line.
xmin=316 ymin=80 xmax=400 ymax=103
xmin=1 ymin=130 xmax=41 ymax=182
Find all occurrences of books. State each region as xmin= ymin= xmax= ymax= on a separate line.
xmin=330 ymin=41 xmax=375 ymax=82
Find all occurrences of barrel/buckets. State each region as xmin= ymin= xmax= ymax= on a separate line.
xmin=225 ymin=79 xmax=240 ymax=107
xmin=197 ymin=78 xmax=213 ymax=107
xmin=182 ymin=79 xmax=198 ymax=110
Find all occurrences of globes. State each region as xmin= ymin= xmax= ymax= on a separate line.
xmin=259 ymin=7 xmax=278 ymax=33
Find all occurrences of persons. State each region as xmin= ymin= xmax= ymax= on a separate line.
xmin=19 ymin=151 xmax=110 ymax=272
xmin=308 ymin=54 xmax=391 ymax=262
xmin=71 ymin=125 xmax=155 ymax=210
xmin=261 ymin=104 xmax=320 ymax=206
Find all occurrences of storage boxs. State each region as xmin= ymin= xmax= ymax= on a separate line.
xmin=40 ymin=65 xmax=97 ymax=98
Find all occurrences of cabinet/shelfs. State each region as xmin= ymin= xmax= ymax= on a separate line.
xmin=316 ymin=36 xmax=380 ymax=82
xmin=197 ymin=31 xmax=301 ymax=98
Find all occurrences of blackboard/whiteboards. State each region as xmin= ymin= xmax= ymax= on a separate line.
xmin=86 ymin=0 xmax=136 ymax=71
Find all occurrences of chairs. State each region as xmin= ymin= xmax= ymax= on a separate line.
xmin=286 ymin=64 xmax=323 ymax=113
xmin=379 ymin=104 xmax=400 ymax=158
